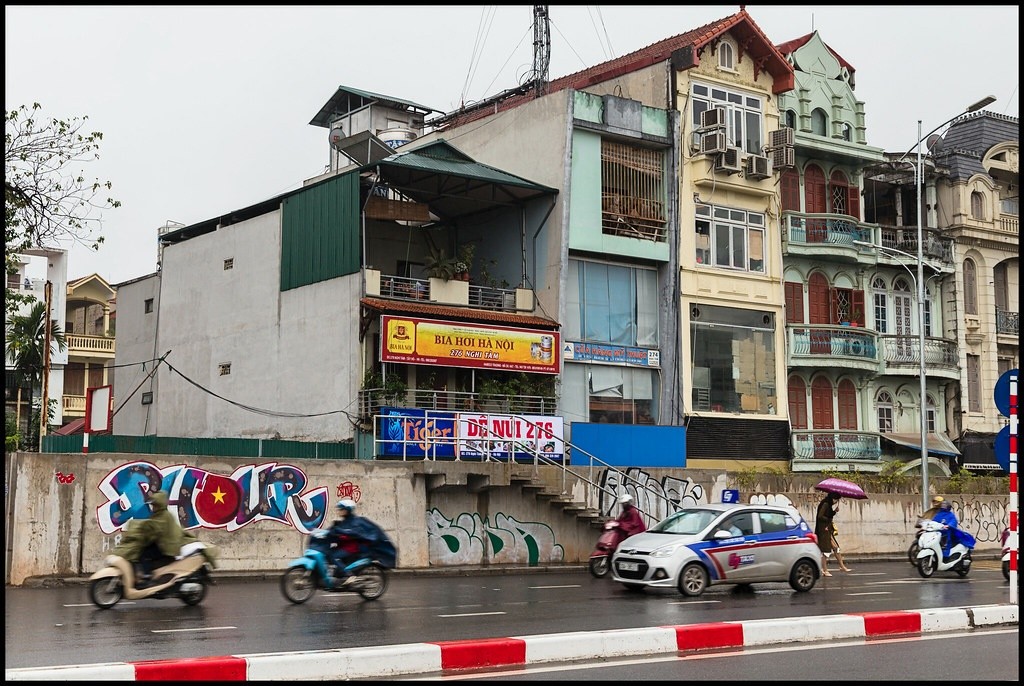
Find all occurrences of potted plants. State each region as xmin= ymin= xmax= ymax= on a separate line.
xmin=457 ymin=242 xmax=478 ymax=281
xmin=360 ymin=362 xmax=410 ymax=416
xmin=420 ymin=247 xmax=457 ymax=283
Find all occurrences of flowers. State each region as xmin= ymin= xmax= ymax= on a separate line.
xmin=453 ymin=261 xmax=468 ymax=274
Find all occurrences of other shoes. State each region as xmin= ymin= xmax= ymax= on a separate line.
xmin=342 ymin=575 xmax=358 ymax=586
xmin=822 ymin=570 xmax=832 ymax=577
xmin=839 ymin=566 xmax=849 ymax=572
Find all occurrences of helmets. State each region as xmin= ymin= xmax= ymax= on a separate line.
xmin=137 ymin=575 xmax=153 ymax=589
xmin=932 ymin=496 xmax=944 ymax=508
xmin=336 ymin=500 xmax=356 ymax=513
xmin=940 ymin=501 xmax=950 ymax=511
xmin=618 ymin=494 xmax=634 ymax=505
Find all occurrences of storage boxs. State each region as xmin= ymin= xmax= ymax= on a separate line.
xmin=731 ymin=332 xmax=775 ymax=410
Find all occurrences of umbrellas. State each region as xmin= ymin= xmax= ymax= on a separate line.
xmin=814 ymin=478 xmax=868 ymax=508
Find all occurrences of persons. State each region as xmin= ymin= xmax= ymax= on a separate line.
xmin=717 ymin=520 xmax=743 ymax=538
xmin=313 ymin=500 xmax=363 ymax=586
xmin=131 ymin=491 xmax=182 ymax=584
xmin=923 ymin=496 xmax=957 ymax=562
xmin=609 ymin=494 xmax=647 ymax=539
xmin=813 ymin=491 xmax=853 ymax=577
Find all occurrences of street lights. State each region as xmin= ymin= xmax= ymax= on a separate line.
xmin=851 ymin=94 xmax=997 ymax=514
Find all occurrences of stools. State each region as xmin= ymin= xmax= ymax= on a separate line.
xmin=711 ymin=404 xmax=722 ymax=411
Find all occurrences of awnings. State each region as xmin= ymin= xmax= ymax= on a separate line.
xmin=880 ymin=433 xmax=962 ymax=456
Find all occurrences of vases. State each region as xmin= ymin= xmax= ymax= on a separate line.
xmin=455 ymin=272 xmax=462 ymax=281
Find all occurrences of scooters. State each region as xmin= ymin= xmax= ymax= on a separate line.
xmin=907 ymin=518 xmax=933 ymax=567
xmin=588 ymin=520 xmax=624 ymax=580
xmin=87 ymin=529 xmax=218 ymax=609
xmin=1001 ymin=527 xmax=1020 ymax=582
xmin=280 ymin=529 xmax=388 ymax=604
xmin=916 ymin=520 xmax=973 ymax=579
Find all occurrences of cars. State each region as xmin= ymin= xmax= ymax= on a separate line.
xmin=611 ymin=503 xmax=822 ymax=599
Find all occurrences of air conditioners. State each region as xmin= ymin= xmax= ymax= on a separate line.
xmin=713 ymin=148 xmax=742 ymax=173
xmin=772 ymin=149 xmax=795 ymax=169
xmin=746 ymin=155 xmax=773 ymax=181
xmin=769 ymin=128 xmax=795 ymax=149
xmin=844 ymin=338 xmax=866 ymax=356
xmin=699 ymin=132 xmax=728 ymax=153
xmin=698 ymin=107 xmax=726 ymax=132
